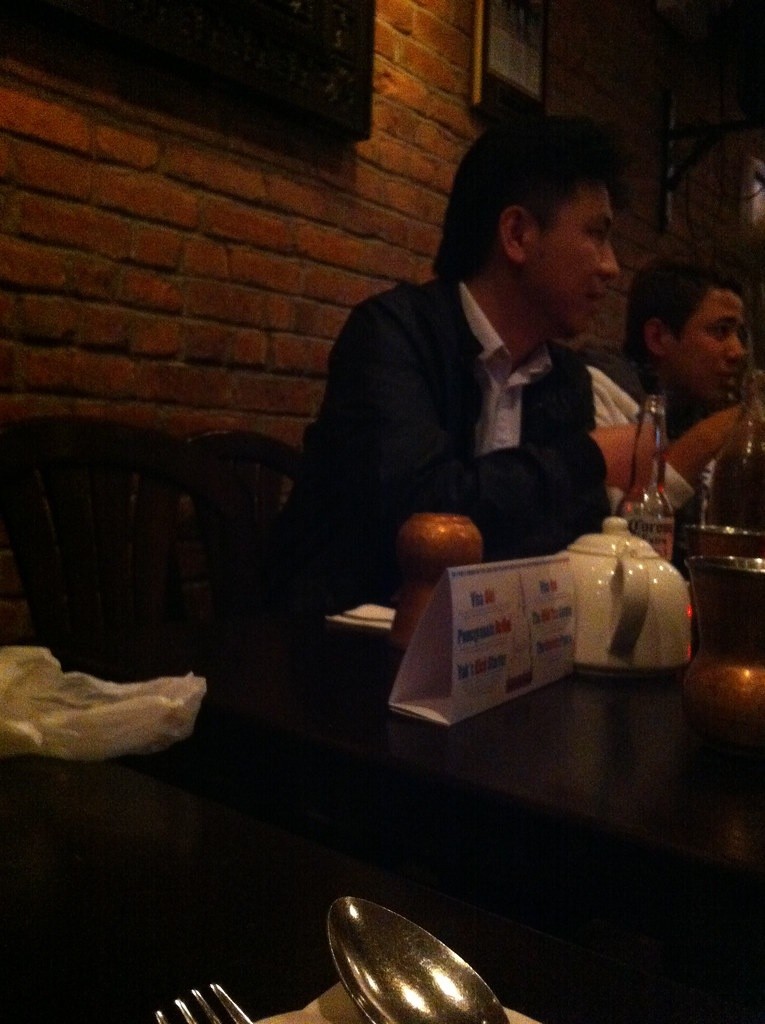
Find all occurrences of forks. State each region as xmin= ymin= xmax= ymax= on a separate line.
xmin=153 ymin=982 xmax=254 ymax=1024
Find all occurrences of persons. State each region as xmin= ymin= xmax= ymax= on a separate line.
xmin=290 ymin=115 xmax=765 ymax=720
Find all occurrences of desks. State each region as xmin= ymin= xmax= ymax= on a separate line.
xmin=0 ymin=761 xmax=765 ymax=1024
xmin=68 ymin=598 xmax=765 ymax=964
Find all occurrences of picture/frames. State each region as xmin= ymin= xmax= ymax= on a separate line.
xmin=472 ymin=0 xmax=550 ymax=127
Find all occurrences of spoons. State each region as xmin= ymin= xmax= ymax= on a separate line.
xmin=327 ymin=896 xmax=510 ymax=1024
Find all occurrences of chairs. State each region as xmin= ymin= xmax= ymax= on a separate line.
xmin=0 ymin=413 xmax=303 ymax=681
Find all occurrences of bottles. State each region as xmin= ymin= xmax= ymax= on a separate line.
xmin=705 ymin=367 xmax=765 ymax=549
xmin=617 ymin=391 xmax=675 ymax=567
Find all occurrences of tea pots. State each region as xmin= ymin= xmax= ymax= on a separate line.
xmin=548 ymin=518 xmax=694 ymax=675
